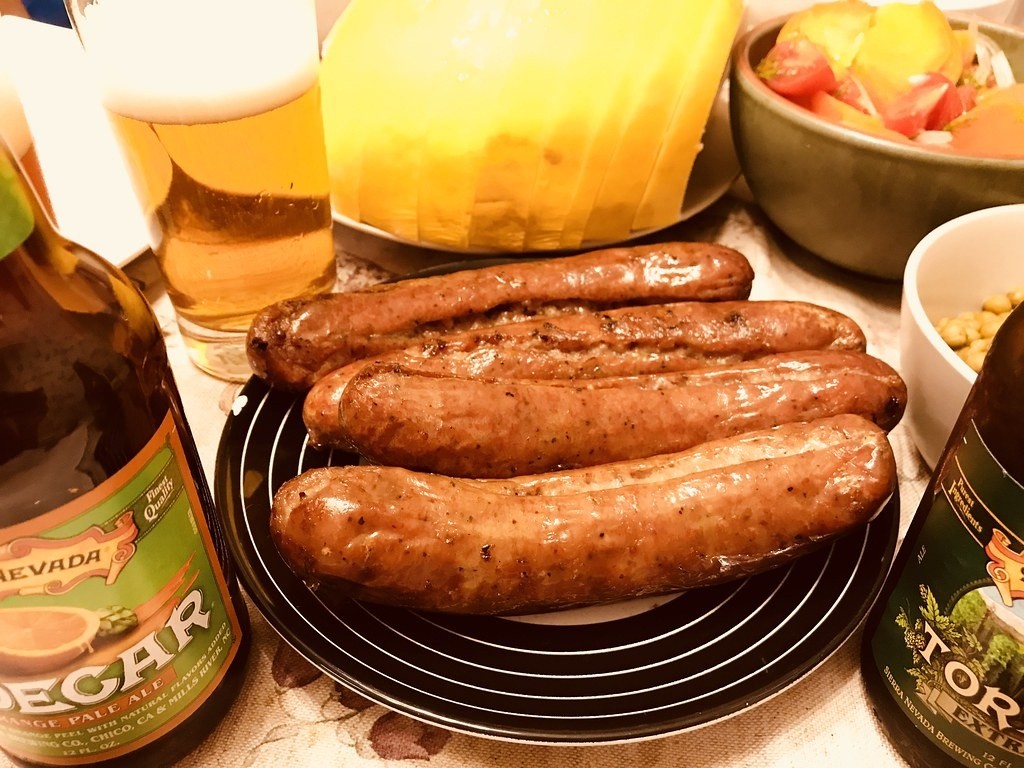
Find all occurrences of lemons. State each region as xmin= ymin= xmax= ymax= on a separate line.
xmin=314 ymin=3 xmax=748 ymax=244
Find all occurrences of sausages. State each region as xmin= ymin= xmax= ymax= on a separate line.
xmin=244 ymin=244 xmax=912 ymax=615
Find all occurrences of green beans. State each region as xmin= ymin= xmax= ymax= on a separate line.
xmin=935 ymin=288 xmax=1024 ymax=373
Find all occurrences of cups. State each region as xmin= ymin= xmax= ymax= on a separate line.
xmin=0 ymin=67 xmax=60 ymax=236
xmin=57 ymin=0 xmax=337 ymax=382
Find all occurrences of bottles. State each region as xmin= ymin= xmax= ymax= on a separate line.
xmin=1 ymin=139 xmax=255 ymax=768
xmin=864 ymin=301 xmax=1024 ymax=767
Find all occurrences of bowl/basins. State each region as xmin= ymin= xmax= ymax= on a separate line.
xmin=728 ymin=6 xmax=1024 ymax=281
xmin=898 ymin=204 xmax=1024 ymax=472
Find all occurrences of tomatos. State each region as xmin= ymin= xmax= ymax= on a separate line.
xmin=758 ymin=0 xmax=1022 ymax=165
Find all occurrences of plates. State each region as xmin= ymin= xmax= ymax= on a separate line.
xmin=332 ymin=94 xmax=743 ymax=275
xmin=212 ymin=354 xmax=900 ymax=747
